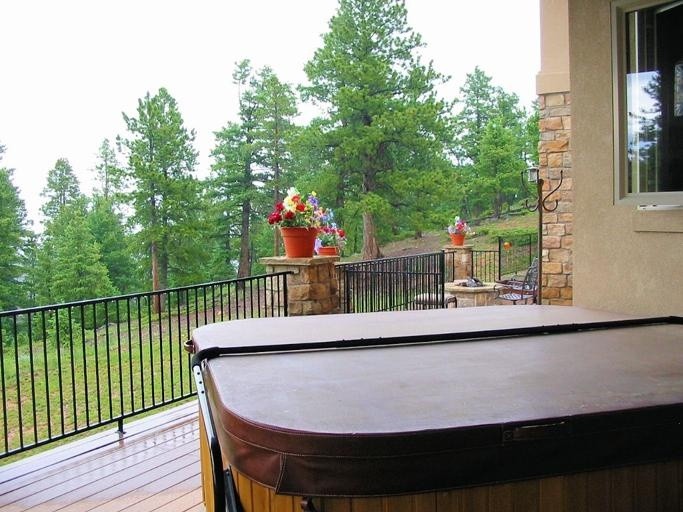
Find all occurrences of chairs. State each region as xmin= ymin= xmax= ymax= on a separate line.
xmin=493 ymin=258 xmax=538 ymax=305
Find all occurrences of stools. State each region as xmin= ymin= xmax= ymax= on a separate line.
xmin=413 ymin=292 xmax=457 ymax=309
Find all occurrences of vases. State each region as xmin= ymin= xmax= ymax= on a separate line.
xmin=449 ymin=233 xmax=465 ymax=246
xmin=281 ymin=227 xmax=339 ymax=258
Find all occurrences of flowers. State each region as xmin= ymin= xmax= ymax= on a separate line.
xmin=266 ymin=187 xmax=345 ymax=247
xmin=447 ymin=216 xmax=471 ymax=235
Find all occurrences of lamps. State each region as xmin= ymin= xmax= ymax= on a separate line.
xmin=519 ymin=163 xmax=541 ymax=200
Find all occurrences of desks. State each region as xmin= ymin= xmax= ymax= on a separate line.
xmin=444 ymin=282 xmax=504 ymax=306
